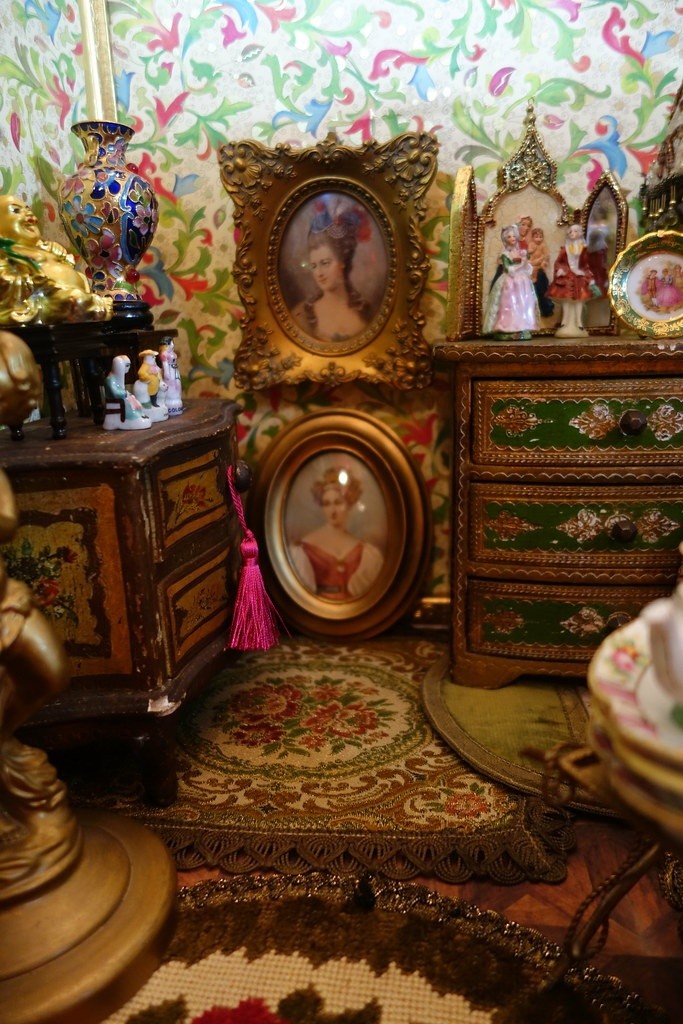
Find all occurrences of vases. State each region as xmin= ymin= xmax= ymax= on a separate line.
xmin=59 ymin=120 xmax=159 ymax=310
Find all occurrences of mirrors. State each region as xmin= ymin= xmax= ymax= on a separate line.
xmin=577 ymin=171 xmax=630 ymax=336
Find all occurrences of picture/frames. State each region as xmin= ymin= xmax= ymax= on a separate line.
xmin=214 ymin=129 xmax=442 ymax=393
xmin=243 ymin=408 xmax=431 ymax=644
xmin=474 ymin=105 xmax=571 ymax=339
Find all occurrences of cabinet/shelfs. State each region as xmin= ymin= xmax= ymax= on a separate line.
xmin=426 ymin=335 xmax=683 ymax=689
xmin=0 ymin=396 xmax=240 ymax=807
